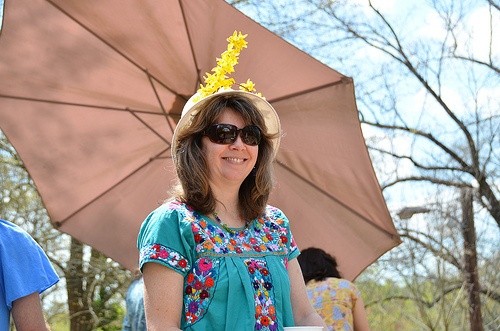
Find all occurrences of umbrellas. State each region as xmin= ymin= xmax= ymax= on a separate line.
xmin=0 ymin=0 xmax=404 ymax=283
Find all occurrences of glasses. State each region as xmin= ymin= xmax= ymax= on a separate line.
xmin=201 ymin=123 xmax=261 ymax=146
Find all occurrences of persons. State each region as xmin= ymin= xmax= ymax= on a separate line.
xmin=121 ymin=277 xmax=148 ymax=331
xmin=297 ymin=247 xmax=370 ymax=331
xmin=137 ymin=30 xmax=329 ymax=331
xmin=0 ymin=218 xmax=60 ymax=331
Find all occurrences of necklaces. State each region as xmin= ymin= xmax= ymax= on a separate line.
xmin=211 ymin=211 xmax=255 ymax=234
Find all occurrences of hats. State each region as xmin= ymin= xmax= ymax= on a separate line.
xmin=170 ymin=31 xmax=282 ymax=176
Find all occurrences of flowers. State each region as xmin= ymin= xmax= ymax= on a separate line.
xmin=188 ymin=28 xmax=267 ymax=104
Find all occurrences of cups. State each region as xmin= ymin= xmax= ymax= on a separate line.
xmin=283 ymin=326 xmax=324 ymax=331
